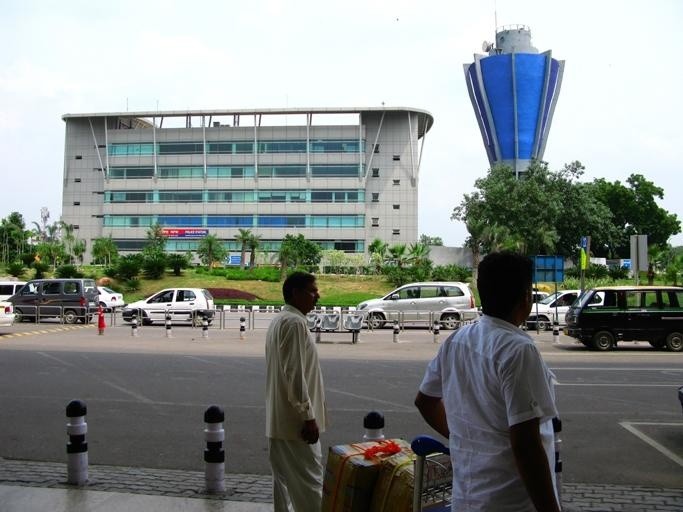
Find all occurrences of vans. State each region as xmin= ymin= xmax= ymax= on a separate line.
xmin=563 ymin=286 xmax=683 ymax=352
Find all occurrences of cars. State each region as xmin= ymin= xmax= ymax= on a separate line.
xmin=520 ymin=288 xmax=605 ymax=331
xmin=0 ymin=277 xmax=127 ymax=331
xmin=122 ymin=286 xmax=215 ymax=328
xmin=355 ymin=281 xmax=478 ymax=331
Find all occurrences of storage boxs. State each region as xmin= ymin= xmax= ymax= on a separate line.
xmin=320 ymin=436 xmax=452 ymax=510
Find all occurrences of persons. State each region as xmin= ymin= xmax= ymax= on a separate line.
xmin=414 ymin=248 xmax=562 ymax=511
xmin=265 ymin=271 xmax=329 ymax=511
xmin=438 ymin=287 xmax=447 ymax=296
xmin=407 ymin=289 xmax=413 ymax=298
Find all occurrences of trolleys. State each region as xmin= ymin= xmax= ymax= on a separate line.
xmin=412 ymin=434 xmax=454 ymax=512
